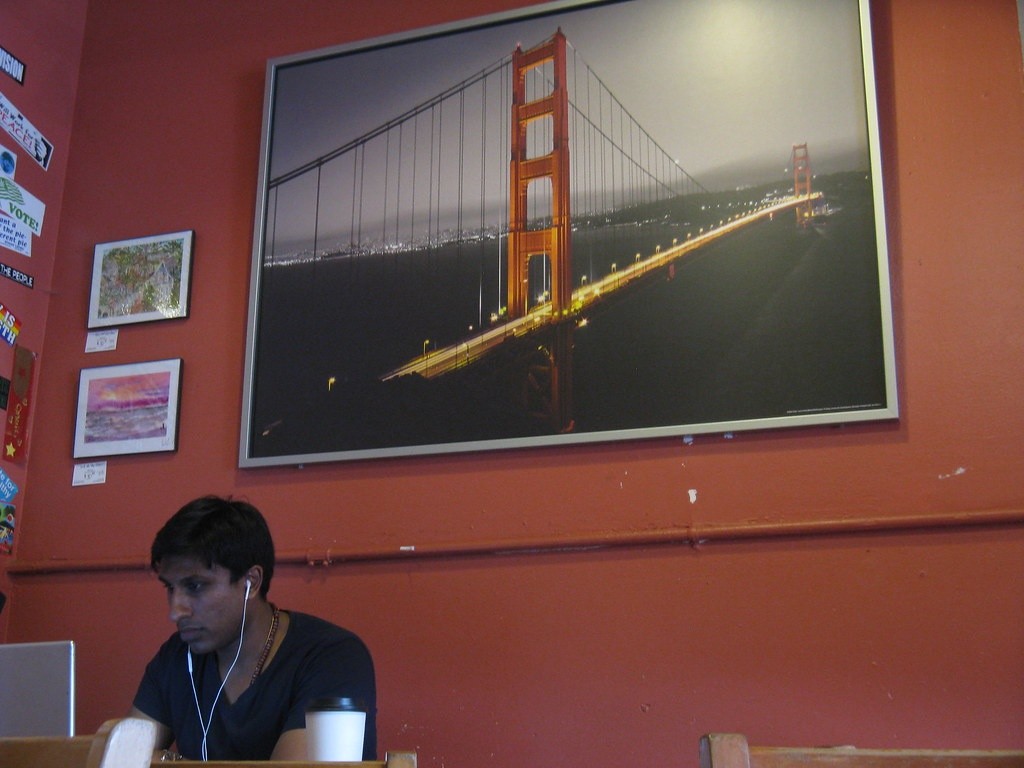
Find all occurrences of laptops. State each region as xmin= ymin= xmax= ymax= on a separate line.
xmin=0 ymin=641 xmax=76 ymax=738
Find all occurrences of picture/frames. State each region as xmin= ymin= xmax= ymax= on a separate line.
xmin=238 ymin=0 xmax=899 ymax=465
xmin=71 ymin=357 xmax=186 ymax=460
xmin=86 ymin=228 xmax=193 ymax=330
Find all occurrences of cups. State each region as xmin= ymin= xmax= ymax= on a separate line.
xmin=303 ymin=690 xmax=368 ymax=762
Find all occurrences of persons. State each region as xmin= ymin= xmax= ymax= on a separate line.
xmin=96 ymin=494 xmax=379 ymax=763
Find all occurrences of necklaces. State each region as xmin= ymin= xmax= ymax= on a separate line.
xmin=249 ymin=604 xmax=281 ymax=687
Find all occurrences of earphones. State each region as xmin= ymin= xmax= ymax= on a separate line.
xmin=244 ymin=579 xmax=252 ymax=600
